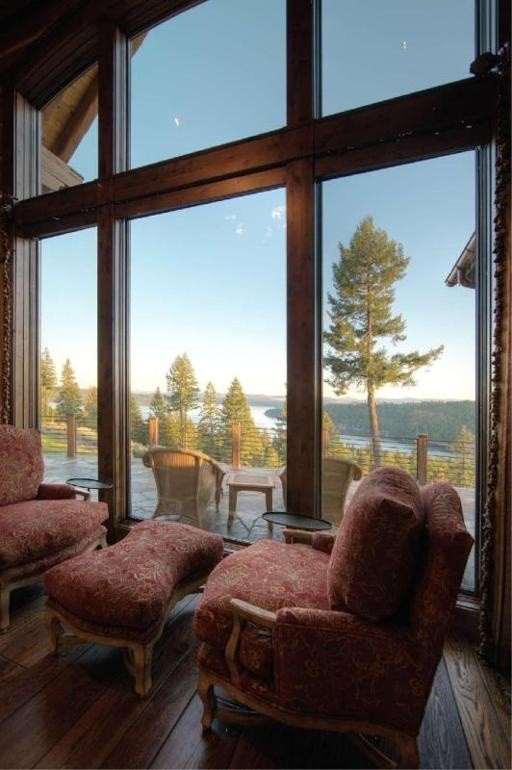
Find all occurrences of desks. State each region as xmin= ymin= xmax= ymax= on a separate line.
xmin=65 ymin=478 xmax=114 ymax=492
xmin=260 ymin=511 xmax=333 ymax=532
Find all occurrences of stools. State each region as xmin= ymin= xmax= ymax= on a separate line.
xmin=44 ymin=518 xmax=225 ymax=700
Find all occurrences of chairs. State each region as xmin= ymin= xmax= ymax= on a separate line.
xmin=279 ymin=453 xmax=361 ymax=534
xmin=0 ymin=425 xmax=111 ymax=635
xmin=196 ymin=465 xmax=475 ymax=767
xmin=144 ymin=448 xmax=225 ymax=532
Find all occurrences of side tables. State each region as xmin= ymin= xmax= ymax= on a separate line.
xmin=227 ymin=472 xmax=275 ymax=538
